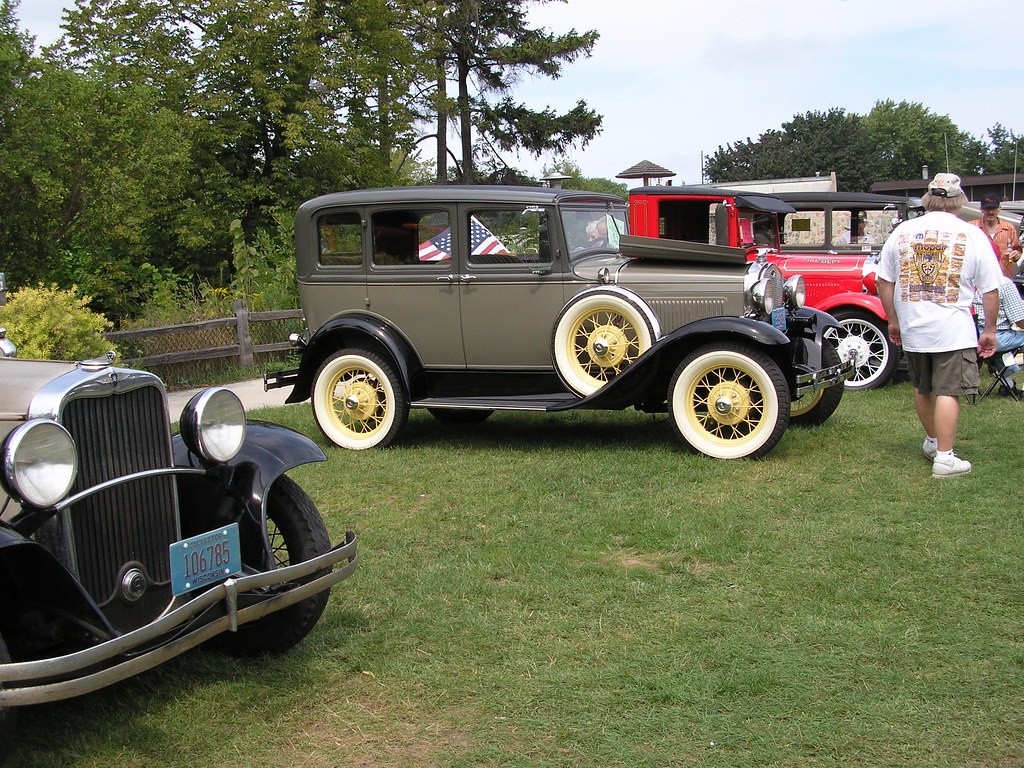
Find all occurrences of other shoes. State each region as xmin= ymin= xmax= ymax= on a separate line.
xmin=998 ymin=382 xmax=1024 ymax=396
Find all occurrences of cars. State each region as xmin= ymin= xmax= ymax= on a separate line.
xmin=0 ymin=330 xmax=359 ymax=721
xmin=905 ymin=195 xmax=1023 ymax=292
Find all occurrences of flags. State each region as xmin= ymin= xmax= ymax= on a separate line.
xmin=419 ymin=216 xmax=511 ymax=261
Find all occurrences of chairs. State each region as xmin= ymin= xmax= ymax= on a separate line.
xmin=963 ymin=313 xmax=1022 ymax=407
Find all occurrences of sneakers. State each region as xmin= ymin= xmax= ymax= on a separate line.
xmin=923 ymin=436 xmax=938 ymax=461
xmin=931 ymin=454 xmax=972 ymax=476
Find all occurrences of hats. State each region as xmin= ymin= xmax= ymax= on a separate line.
xmin=981 ymin=193 xmax=999 ymax=208
xmin=991 ymin=243 xmax=1001 ymax=262
xmin=928 ymin=173 xmax=964 ymax=198
xmin=858 ymin=211 xmax=867 ymax=220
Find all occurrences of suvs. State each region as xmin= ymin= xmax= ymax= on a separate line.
xmin=626 ymin=181 xmax=926 ymax=395
xmin=263 ymin=186 xmax=857 ymax=463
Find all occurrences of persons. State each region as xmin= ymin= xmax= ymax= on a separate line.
xmin=586 ymin=217 xmax=609 ymax=247
xmin=965 ymin=192 xmax=1023 ymax=280
xmin=838 ymin=211 xmax=874 ymax=244
xmin=877 ymin=173 xmax=1004 ymax=478
xmin=973 ymin=275 xmax=1024 ymax=397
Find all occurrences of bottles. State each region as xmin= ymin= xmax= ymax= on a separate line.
xmin=1006 ymin=238 xmax=1013 ymax=263
